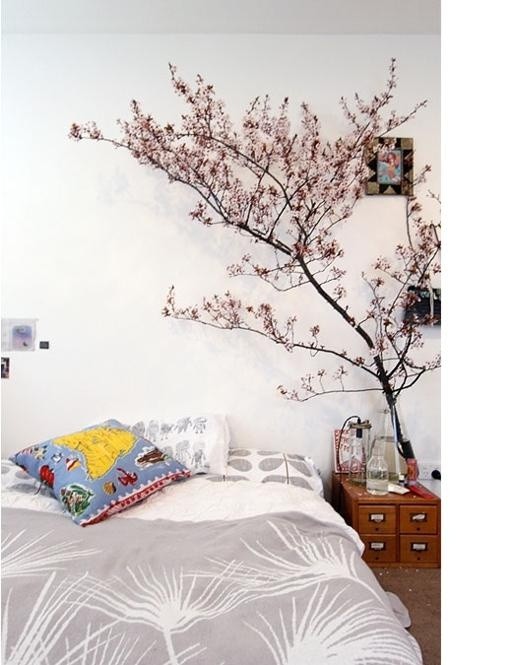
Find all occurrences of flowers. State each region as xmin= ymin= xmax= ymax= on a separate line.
xmin=66 ymin=56 xmax=442 ymax=447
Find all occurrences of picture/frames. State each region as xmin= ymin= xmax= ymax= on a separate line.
xmin=359 ymin=135 xmax=414 ymax=198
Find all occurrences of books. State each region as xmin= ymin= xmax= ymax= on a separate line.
xmin=333 ymin=428 xmax=366 ymax=473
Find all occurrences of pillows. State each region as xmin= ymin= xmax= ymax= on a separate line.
xmin=7 ymin=409 xmax=230 ymax=527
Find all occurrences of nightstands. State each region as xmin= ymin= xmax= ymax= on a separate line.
xmin=332 ymin=473 xmax=441 ymax=570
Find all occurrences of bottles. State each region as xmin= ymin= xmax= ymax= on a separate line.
xmin=349 ymin=408 xmax=401 ymax=497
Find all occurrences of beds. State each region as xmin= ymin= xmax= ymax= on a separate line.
xmin=3 ymin=450 xmax=425 ymax=665
